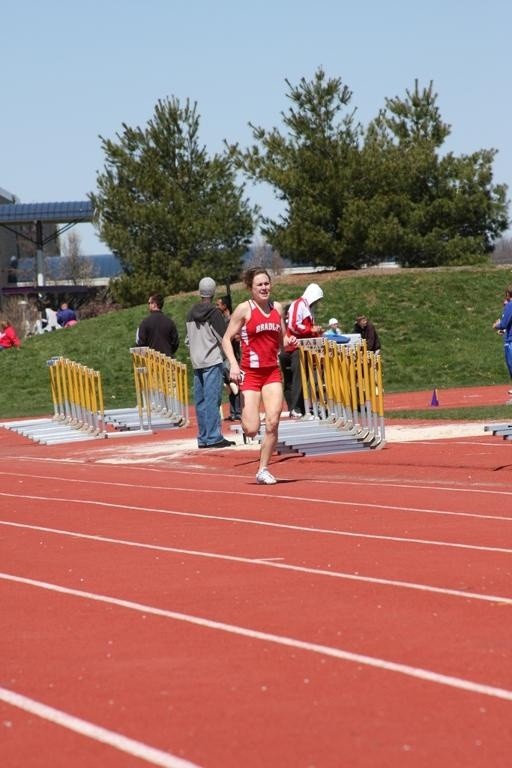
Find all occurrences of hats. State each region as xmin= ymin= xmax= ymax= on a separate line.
xmin=328 ymin=318 xmax=338 ymax=326
xmin=200 ymin=276 xmax=216 ymax=297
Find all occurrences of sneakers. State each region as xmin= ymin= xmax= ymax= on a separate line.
xmin=223 ymin=413 xmax=236 ymax=422
xmin=234 ymin=412 xmax=241 ymax=421
xmin=255 ymin=465 xmax=277 ymax=485
xmin=290 ymin=409 xmax=302 ymax=417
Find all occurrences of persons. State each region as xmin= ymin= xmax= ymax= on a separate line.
xmin=134 ymin=294 xmax=179 ymax=359
xmin=215 ymin=266 xmax=381 ymax=484
xmin=492 ymin=286 xmax=512 ymax=395
xmin=184 ymin=277 xmax=236 ymax=449
xmin=0 ymin=303 xmax=76 ymax=349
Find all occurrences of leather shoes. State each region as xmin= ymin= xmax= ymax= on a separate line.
xmin=207 ymin=440 xmax=236 ymax=448
